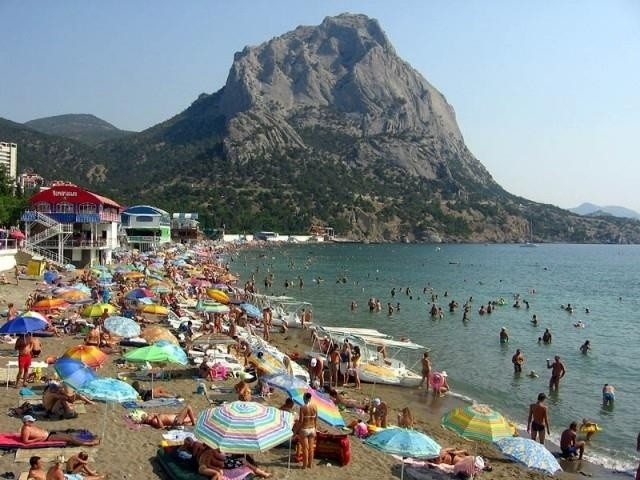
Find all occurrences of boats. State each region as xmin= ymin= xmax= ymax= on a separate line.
xmin=240 ymin=291 xmax=315 ymax=329
xmin=518 ymin=241 xmax=537 ymax=247
xmin=303 ymin=321 xmax=423 ymax=389
xmin=203 ymin=287 xmax=230 ymax=305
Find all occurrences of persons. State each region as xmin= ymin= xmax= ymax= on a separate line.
xmin=526 ymin=391 xmax=550 ymax=446
xmin=434 ymin=369 xmax=451 ymax=398
xmin=42 ymin=384 xmax=94 ymax=413
xmin=418 ymin=351 xmax=433 ymax=393
xmin=585 ymin=308 xmax=590 ymax=314
xmin=565 ymin=304 xmax=574 ymax=313
xmin=543 ymin=329 xmax=553 ymax=344
xmin=545 ymin=353 xmax=566 ymax=391
xmin=578 ymin=417 xmax=599 ymax=442
xmin=530 ymin=314 xmax=540 ymax=328
xmin=601 ymin=383 xmax=615 ymax=410
xmin=524 ymin=370 xmax=539 ymax=378
xmin=430 ymin=447 xmax=475 ymax=467
xmin=578 ymin=340 xmax=592 ymax=355
xmin=185 ymin=438 xmax=272 ymax=480
xmin=537 ymin=337 xmax=543 ymax=344
xmin=560 ymin=422 xmax=586 ymax=461
xmin=26 ymin=452 xmax=104 ymax=480
xmin=141 ymin=405 xmax=197 ymax=428
xmin=421 ymin=276 xmax=541 ymax=324
xmin=331 ymin=389 xmax=411 ymax=437
xmin=511 ymin=348 xmax=525 ymax=373
xmin=499 ymin=327 xmax=512 ymax=344
xmin=21 ymin=415 xmax=100 ymax=446
xmin=282 ymin=393 xmax=318 ymax=469
xmin=574 ymin=319 xmax=585 ymax=328
xmin=0 ymin=225 xmax=417 ymax=402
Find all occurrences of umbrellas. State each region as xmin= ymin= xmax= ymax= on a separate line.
xmin=363 ymin=427 xmax=442 ymax=480
xmin=485 ymin=436 xmax=564 ymax=479
xmin=438 ymin=404 xmax=517 ymax=462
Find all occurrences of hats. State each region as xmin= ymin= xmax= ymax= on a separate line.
xmin=23 ymin=415 xmax=35 ymax=422
xmin=311 ymin=358 xmax=316 ymax=367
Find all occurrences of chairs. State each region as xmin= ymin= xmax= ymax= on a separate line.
xmin=167 ymin=310 xmax=243 ymax=379
